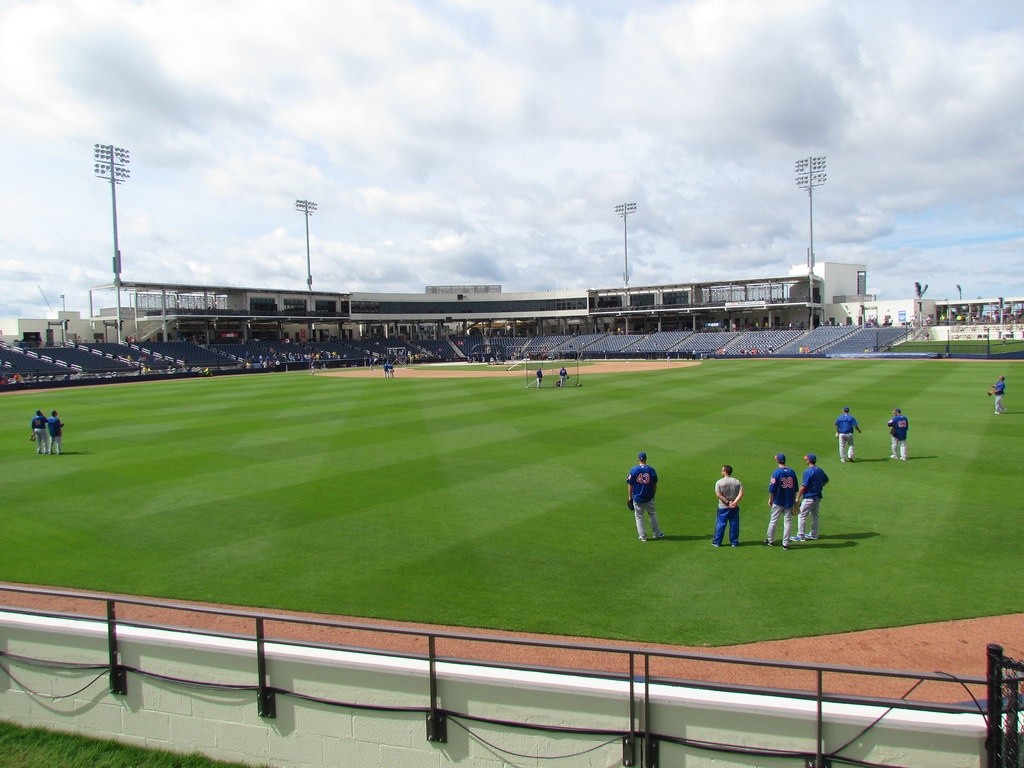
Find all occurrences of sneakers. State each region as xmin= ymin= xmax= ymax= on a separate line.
xmin=789 ymin=535 xmax=806 ymax=541
xmin=805 ymin=534 xmax=818 ymax=540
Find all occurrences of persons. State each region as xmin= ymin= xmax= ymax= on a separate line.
xmin=330 ymin=350 xmax=336 ymax=359
xmin=820 ymin=320 xmax=832 ymax=326
xmin=537 ymin=368 xmax=543 ymax=388
xmin=14 ymin=374 xmax=22 ymax=383
xmin=143 ymin=365 xmax=152 ymax=374
xmin=369 ymin=356 xmax=378 ymax=371
xmin=48 ymin=411 xmax=64 ymax=455
xmin=666 ymin=351 xmax=671 ymax=365
xmin=511 ymin=350 xmax=518 ymax=361
xmin=389 ymin=349 xmax=438 ymax=365
xmin=765 ymin=453 xmax=829 ymax=550
xmin=1 ymin=373 xmax=9 ymax=384
xmin=490 ymin=352 xmax=500 ymax=365
xmin=205 ymin=367 xmax=209 ymax=375
xmin=888 ymin=408 xmax=909 ymax=460
xmin=627 ymin=453 xmax=664 ymax=541
xmin=866 ymin=316 xmax=889 ymax=328
xmin=127 ymin=353 xmax=134 ymax=366
xmin=32 ymin=410 xmax=49 ymax=454
xmin=712 ymin=465 xmax=744 ymax=546
xmin=236 ymin=345 xmax=322 ymax=375
xmin=835 ymin=407 xmax=861 ymax=463
xmin=686 ymin=350 xmax=696 ymax=360
xmin=560 ymin=367 xmax=568 ymax=386
xmin=922 ymin=313 xmax=963 ymax=341
xmin=384 ymin=362 xmax=394 ymax=378
xmin=468 ymin=351 xmax=485 ymax=362
xmin=715 ymin=346 xmax=774 ymax=355
xmin=988 ymin=376 xmax=1006 ymax=414
xmin=723 ymin=318 xmax=804 ymax=332
xmin=128 ymin=337 xmax=132 ymax=347
xmin=800 ymin=345 xmax=809 ymax=354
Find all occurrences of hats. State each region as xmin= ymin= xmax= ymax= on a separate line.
xmin=51 ymin=410 xmax=56 ymax=415
xmin=36 ymin=410 xmax=43 ymax=415
xmin=637 ymin=452 xmax=647 ymax=461
xmin=775 ymin=453 xmax=786 ymax=462
xmin=841 ymin=406 xmax=849 ymax=412
xmin=803 ymin=454 xmax=817 ymax=462
xmin=894 ymin=408 xmax=901 ymax=414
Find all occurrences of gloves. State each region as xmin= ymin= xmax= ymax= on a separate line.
xmin=795 ymin=496 xmax=800 ymax=505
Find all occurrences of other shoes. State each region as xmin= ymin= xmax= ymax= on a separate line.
xmin=848 ymin=455 xmax=853 ymax=462
xmin=49 ymin=451 xmax=54 ymax=455
xmin=890 ymin=454 xmax=899 ymax=460
xmin=899 ymin=456 xmax=907 ymax=461
xmin=995 ymin=411 xmax=1001 ymax=415
xmin=731 ymin=543 xmax=737 ymax=547
xmin=653 ymin=532 xmax=664 ymax=538
xmin=840 ymin=459 xmax=846 ymax=463
xmin=782 ymin=545 xmax=788 ymax=550
xmin=57 ymin=453 xmax=62 ymax=455
xmin=638 ymin=536 xmax=647 ymax=542
xmin=764 ymin=540 xmax=773 ymax=546
xmin=711 ymin=542 xmax=720 ymax=547
xmin=44 ymin=451 xmax=48 ymax=454
xmin=1001 ymin=408 xmax=1007 ymax=413
xmin=37 ymin=451 xmax=42 ymax=454
